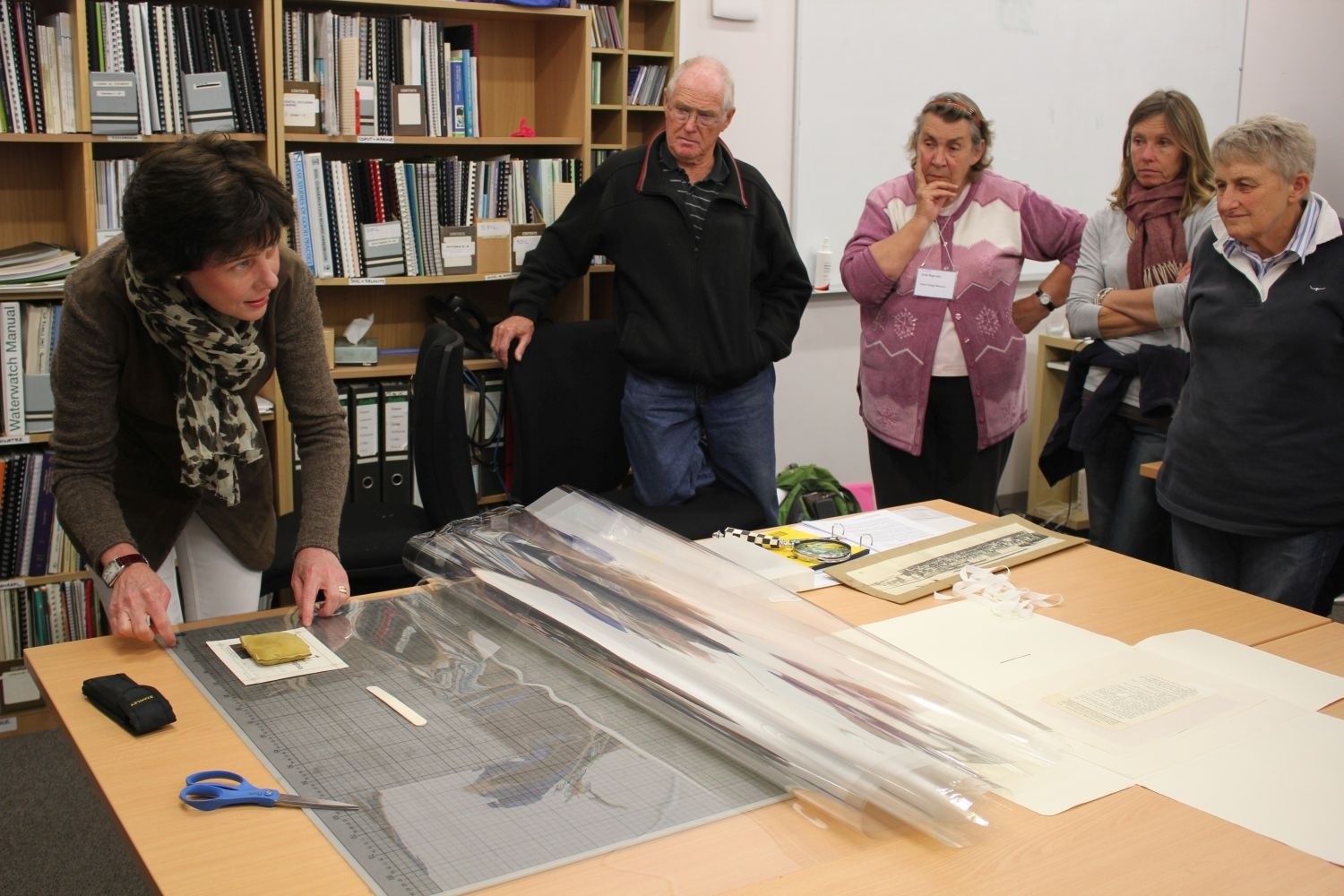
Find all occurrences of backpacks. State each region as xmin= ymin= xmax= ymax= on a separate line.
xmin=777 ymin=462 xmax=862 ymax=527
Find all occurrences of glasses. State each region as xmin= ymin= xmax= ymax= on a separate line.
xmin=664 ymin=96 xmax=729 ymax=127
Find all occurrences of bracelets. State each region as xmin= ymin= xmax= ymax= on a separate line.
xmin=1094 ymin=287 xmax=1114 ymax=306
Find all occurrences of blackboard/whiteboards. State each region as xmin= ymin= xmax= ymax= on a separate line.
xmin=790 ymin=0 xmax=1248 ymax=296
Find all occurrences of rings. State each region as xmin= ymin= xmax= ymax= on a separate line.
xmin=338 ymin=585 xmax=347 ymax=594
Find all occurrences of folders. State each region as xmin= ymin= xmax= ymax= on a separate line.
xmin=293 ymin=376 xmax=413 ymax=504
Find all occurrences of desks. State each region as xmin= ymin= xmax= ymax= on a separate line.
xmin=1023 ymin=332 xmax=1096 ymax=531
xmin=21 ymin=496 xmax=1344 ymax=896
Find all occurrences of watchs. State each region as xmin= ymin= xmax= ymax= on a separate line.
xmin=1035 ymin=289 xmax=1058 ymax=312
xmin=99 ymin=553 xmax=149 ymax=589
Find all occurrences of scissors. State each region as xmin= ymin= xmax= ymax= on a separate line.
xmin=179 ymin=771 xmax=360 ymax=811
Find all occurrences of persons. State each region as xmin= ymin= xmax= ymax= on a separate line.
xmin=1065 ymin=89 xmax=1222 ymax=573
xmin=1153 ymin=117 xmax=1344 ymax=626
xmin=838 ymin=92 xmax=1089 ymax=515
xmin=489 ymin=52 xmax=813 ymax=542
xmin=50 ymin=131 xmax=350 ymax=648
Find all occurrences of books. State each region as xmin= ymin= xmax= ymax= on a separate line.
xmin=0 ymin=2 xmax=672 ymax=668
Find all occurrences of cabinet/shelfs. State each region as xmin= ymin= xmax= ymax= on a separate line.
xmin=0 ymin=0 xmax=682 ymax=738
